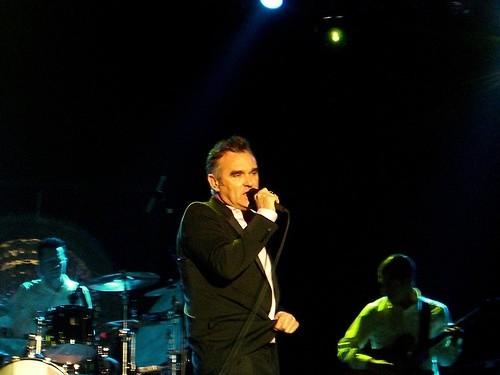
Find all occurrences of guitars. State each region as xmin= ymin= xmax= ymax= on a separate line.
xmin=350 ymin=290 xmax=496 ymax=375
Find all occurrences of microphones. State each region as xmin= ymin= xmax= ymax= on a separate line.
xmin=248 ymin=188 xmax=285 ymax=212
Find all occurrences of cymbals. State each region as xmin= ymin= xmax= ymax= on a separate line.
xmin=87 ymin=272 xmax=161 ymax=293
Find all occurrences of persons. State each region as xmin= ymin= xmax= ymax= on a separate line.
xmin=337 ymin=254 xmax=465 ymax=375
xmin=7 ymin=237 xmax=93 ymax=339
xmin=175 ymin=136 xmax=299 ymax=375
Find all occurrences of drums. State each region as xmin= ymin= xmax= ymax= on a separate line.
xmin=42 ymin=306 xmax=98 ymax=364
xmin=0 ymin=356 xmax=79 ymax=375
